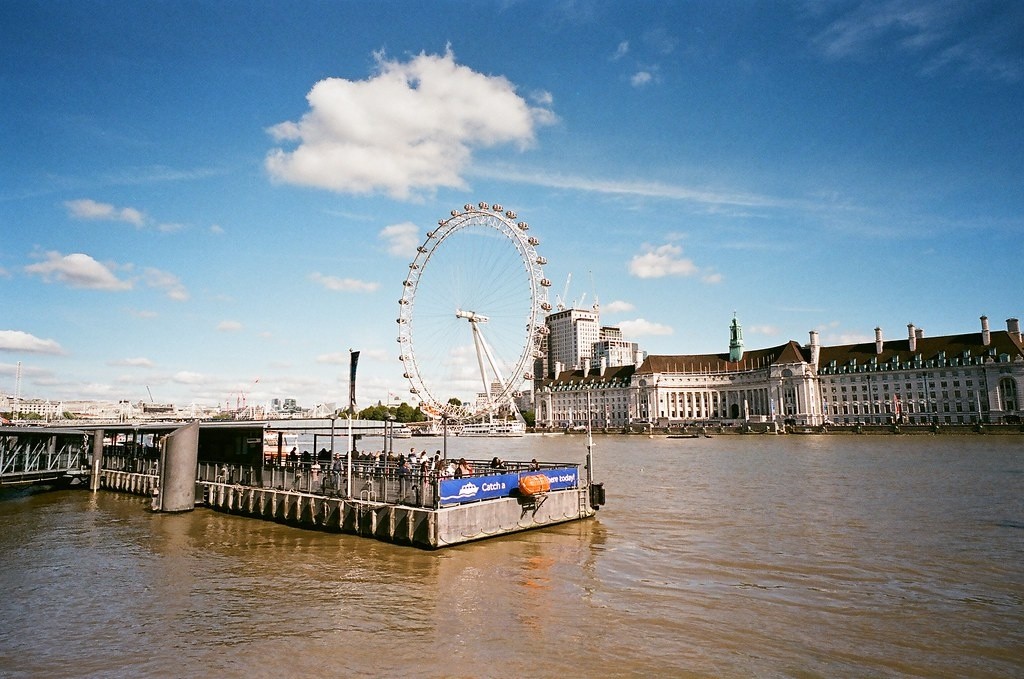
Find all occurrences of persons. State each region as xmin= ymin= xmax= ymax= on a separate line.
xmin=0 ymin=436 xmax=89 ymax=470
xmin=290 ymin=447 xmax=541 ymax=503
xmin=408 ymin=423 xmax=464 ymax=433
xmin=595 ymin=418 xmax=1024 ymax=428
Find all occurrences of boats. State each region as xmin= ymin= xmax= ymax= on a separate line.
xmin=666 ymin=432 xmax=699 ymax=438
xmin=408 ymin=421 xmax=526 ymax=436
xmin=264 ymin=422 xmax=299 ymax=459
xmin=382 ymin=426 xmax=412 ymax=438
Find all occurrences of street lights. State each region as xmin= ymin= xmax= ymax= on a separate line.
xmin=388 ymin=415 xmax=397 ymax=451
xmin=383 ymin=412 xmax=391 ymax=501
xmin=328 ymin=414 xmax=338 ymax=465
xmin=442 ymin=413 xmax=448 ymax=465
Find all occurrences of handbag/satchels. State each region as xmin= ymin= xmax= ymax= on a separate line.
xmin=454 ymin=464 xmax=462 ymax=479
